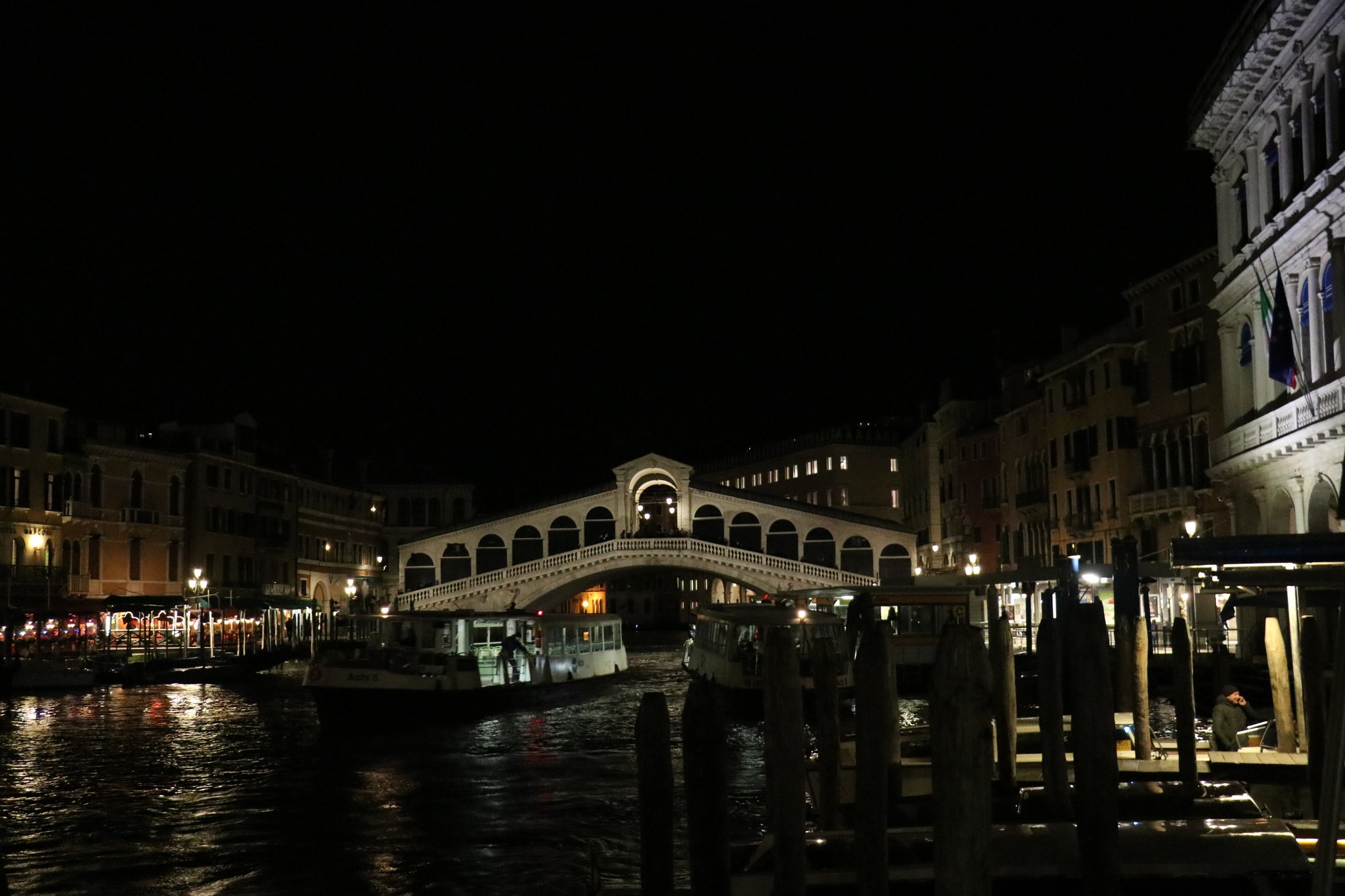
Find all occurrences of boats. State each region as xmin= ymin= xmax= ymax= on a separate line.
xmin=256 ymin=606 xmax=632 ymax=693
xmin=684 ymin=600 xmax=861 ymax=706
xmin=805 ymin=713 xmax=1164 ymax=811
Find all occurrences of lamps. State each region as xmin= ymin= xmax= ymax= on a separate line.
xmin=644 ymin=512 xmax=650 ymax=520
xmin=638 ymin=504 xmax=643 ymax=511
xmin=666 ymin=496 xmax=672 ymax=505
xmin=669 ymin=504 xmax=675 ymax=514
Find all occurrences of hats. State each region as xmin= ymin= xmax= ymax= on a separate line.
xmin=1223 ymin=685 xmax=1239 ymax=697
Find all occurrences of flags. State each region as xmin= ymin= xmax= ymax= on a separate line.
xmin=1254 ymin=269 xmax=1303 ymax=393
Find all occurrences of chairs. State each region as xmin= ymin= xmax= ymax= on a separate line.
xmin=475 ymin=643 xmax=536 ymax=685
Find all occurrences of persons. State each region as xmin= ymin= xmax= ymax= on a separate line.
xmin=502 ymin=632 xmax=533 ymax=683
xmin=575 ymin=521 xmax=765 ymax=565
xmin=1151 ymin=612 xmax=1261 ymax=799
xmin=284 ymin=616 xmax=319 ymax=641
xmin=1001 ymin=606 xmax=1009 ymax=621
